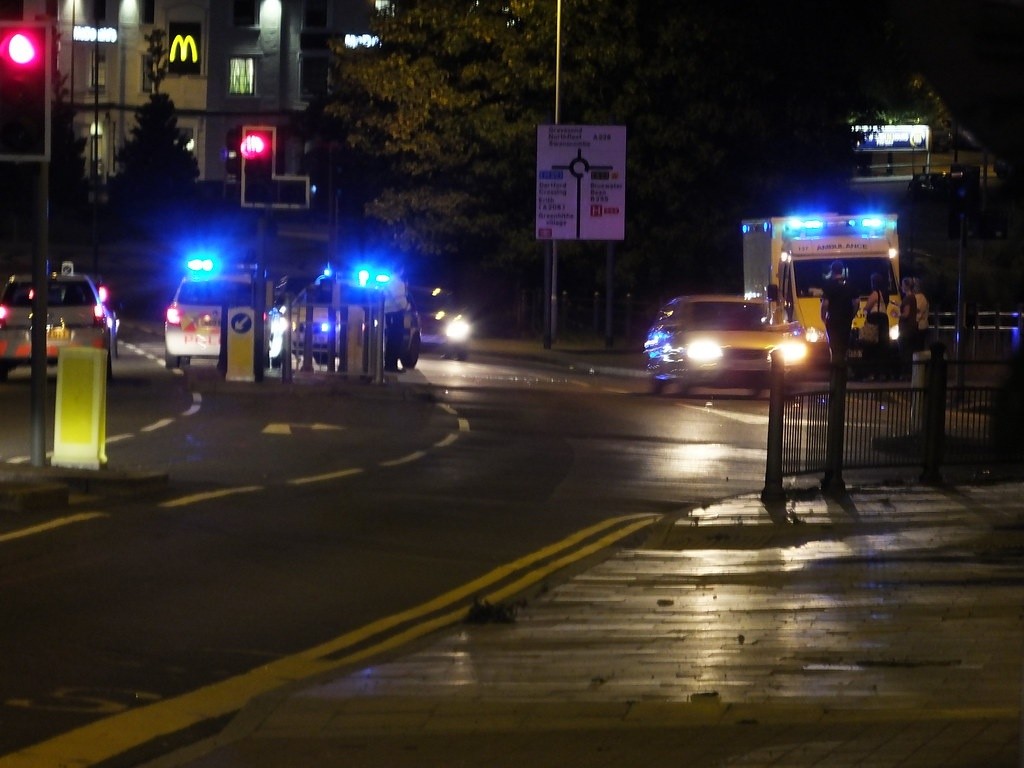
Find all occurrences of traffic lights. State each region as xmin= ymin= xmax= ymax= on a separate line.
xmin=240 ymin=124 xmax=310 ymax=211
xmin=0 ymin=20 xmax=52 ymax=164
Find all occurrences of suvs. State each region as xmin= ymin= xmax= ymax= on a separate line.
xmin=0 ymin=272 xmax=115 ymax=385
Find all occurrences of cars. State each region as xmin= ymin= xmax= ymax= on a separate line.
xmin=409 ymin=285 xmax=470 ymax=365
xmin=165 ymin=257 xmax=288 ymax=372
xmin=645 ymin=294 xmax=803 ymax=401
xmin=285 ymin=262 xmax=422 ymax=368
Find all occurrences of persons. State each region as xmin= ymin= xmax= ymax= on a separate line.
xmin=863 ymin=273 xmax=928 ymax=381
xmin=820 ymin=261 xmax=861 ymax=384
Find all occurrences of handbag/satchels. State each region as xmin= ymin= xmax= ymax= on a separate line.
xmin=858 ymin=320 xmax=879 ymax=343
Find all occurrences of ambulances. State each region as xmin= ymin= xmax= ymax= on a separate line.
xmin=741 ymin=216 xmax=903 ymax=365
